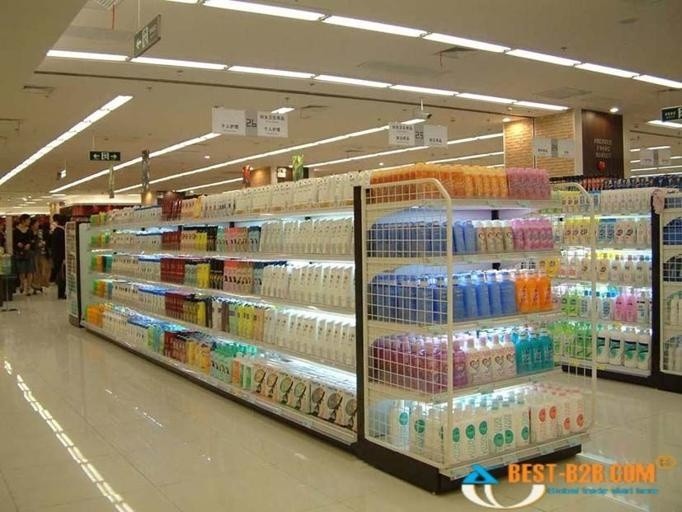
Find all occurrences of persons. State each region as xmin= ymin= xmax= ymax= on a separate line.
xmin=29 ymin=218 xmax=47 ymax=295
xmin=48 ymin=215 xmax=69 ymax=299
xmin=14 ymin=213 xmax=35 ymax=297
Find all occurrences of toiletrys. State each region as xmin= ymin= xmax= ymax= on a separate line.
xmin=80 ymin=155 xmax=682 ymax=462
